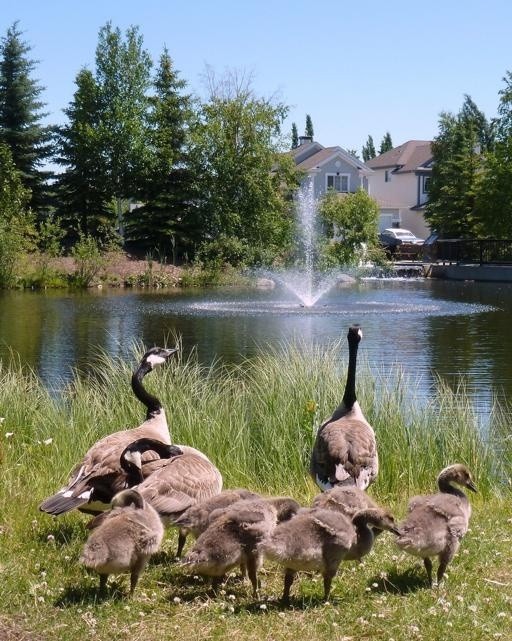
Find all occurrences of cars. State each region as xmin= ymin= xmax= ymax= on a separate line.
xmin=382 ymin=228 xmax=425 ymax=249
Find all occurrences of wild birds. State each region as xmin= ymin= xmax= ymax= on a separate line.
xmin=112 ymin=438 xmax=222 ymax=560
xmin=393 ymin=462 xmax=482 ymax=591
xmin=75 ymin=488 xmax=164 ymax=600
xmin=308 ymin=322 xmax=380 ymax=491
xmin=170 ymin=486 xmax=406 ymax=605
xmin=37 ymin=347 xmax=181 ymax=517
